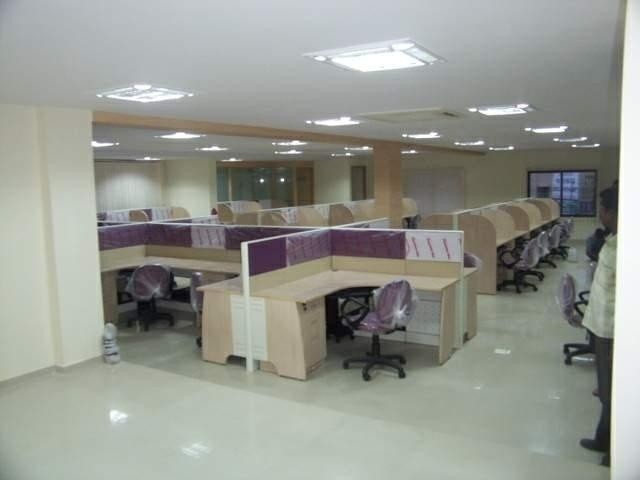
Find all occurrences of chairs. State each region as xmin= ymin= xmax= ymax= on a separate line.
xmin=125 ymin=264 xmax=175 ymax=331
xmin=560 ymin=272 xmax=597 ymax=365
xmin=190 ymin=272 xmax=208 ymax=348
xmin=496 ymin=217 xmax=575 ymax=294
xmin=343 ymin=279 xmax=416 ymax=380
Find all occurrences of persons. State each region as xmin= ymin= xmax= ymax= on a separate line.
xmin=580 ymin=188 xmax=618 ymax=476
xmin=586 ymin=229 xmax=606 ymax=267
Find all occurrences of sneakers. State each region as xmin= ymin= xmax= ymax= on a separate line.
xmin=580 ymin=438 xmax=606 ymax=452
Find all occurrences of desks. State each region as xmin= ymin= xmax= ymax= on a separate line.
xmin=250 ymin=268 xmax=462 ymax=381
xmin=100 ymin=255 xmax=245 ymax=365
